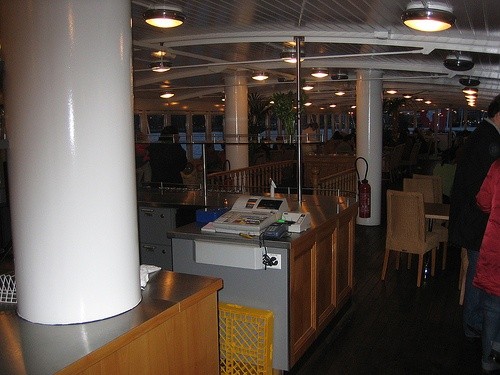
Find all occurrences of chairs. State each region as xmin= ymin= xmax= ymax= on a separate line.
xmin=326 ymin=130 xmax=469 ymax=305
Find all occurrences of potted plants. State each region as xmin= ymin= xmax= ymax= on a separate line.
xmin=247 ymin=94 xmax=270 ymax=142
xmin=270 ymin=91 xmax=306 ymax=142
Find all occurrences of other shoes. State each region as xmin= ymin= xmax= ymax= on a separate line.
xmin=482 ymin=356 xmax=500 ymax=371
xmin=465 ymin=327 xmax=481 ymax=337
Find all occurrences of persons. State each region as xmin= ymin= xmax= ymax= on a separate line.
xmin=149 ymin=125 xmax=188 ymax=192
xmin=448 ymin=94 xmax=500 ymax=375
xmin=303 ymin=122 xmax=319 ymax=142
xmin=329 ymin=124 xmax=428 ymax=157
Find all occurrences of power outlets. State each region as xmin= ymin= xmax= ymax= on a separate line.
xmin=264 ymin=253 xmax=281 ymax=270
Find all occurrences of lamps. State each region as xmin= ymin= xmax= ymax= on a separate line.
xmin=401 ymin=0 xmax=456 ymax=32
xmin=143 ymin=3 xmax=187 ymax=28
xmin=154 ymin=37 xmax=480 ymax=107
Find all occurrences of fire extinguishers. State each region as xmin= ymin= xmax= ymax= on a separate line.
xmin=354 ymin=157 xmax=371 ymax=218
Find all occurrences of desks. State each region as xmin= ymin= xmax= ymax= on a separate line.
xmin=422 ymin=203 xmax=467 ymax=275
